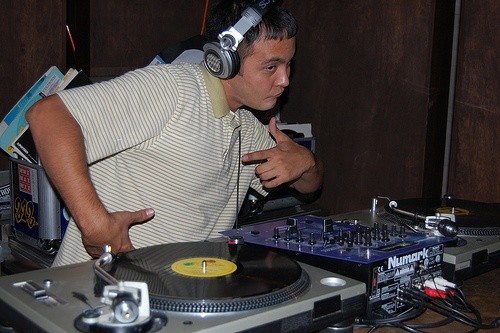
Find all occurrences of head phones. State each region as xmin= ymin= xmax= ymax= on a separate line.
xmin=203 ymin=0 xmax=279 ymax=81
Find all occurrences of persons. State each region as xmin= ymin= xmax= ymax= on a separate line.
xmin=25 ymin=0 xmax=321 ymax=269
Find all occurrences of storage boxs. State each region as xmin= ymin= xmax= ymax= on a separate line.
xmin=239 ymin=135 xmax=317 ymax=220
xmin=8 ymin=155 xmax=72 ymax=257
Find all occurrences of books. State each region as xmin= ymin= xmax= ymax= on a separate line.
xmin=0 ymin=66 xmax=85 ymax=166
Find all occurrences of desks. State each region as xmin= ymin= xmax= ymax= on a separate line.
xmin=313 ymin=266 xmax=500 ymax=333
